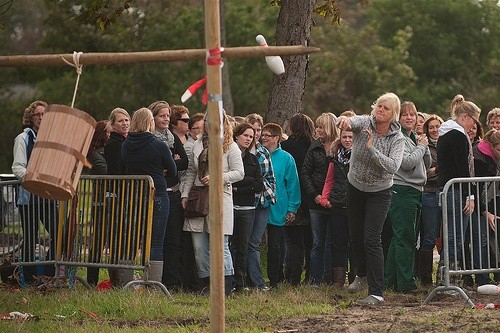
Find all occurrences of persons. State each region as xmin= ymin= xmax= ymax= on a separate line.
xmin=281 ymin=110 xmax=368 ymax=288
xmin=12 ymin=101 xmax=68 ymax=276
xmin=382 ymin=95 xmax=500 ymax=294
xmin=150 ymin=101 xmax=301 ymax=295
xmin=87 ymin=108 xmax=130 ymax=285
xmin=336 ymin=92 xmax=405 ymax=305
xmin=120 ymin=107 xmax=177 ymax=270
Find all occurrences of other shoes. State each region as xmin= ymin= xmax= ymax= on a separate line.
xmin=347 ymin=274 xmax=368 ymax=294
xmin=355 ymin=296 xmax=384 ymax=306
xmin=257 ymin=284 xmax=270 ymax=291
xmin=407 ymin=287 xmax=427 ymax=292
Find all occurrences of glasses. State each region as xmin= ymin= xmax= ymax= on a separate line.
xmin=467 ymin=113 xmax=479 ymax=127
xmin=261 ymin=134 xmax=277 ymax=139
xmin=177 ymin=117 xmax=190 ymax=124
xmin=32 ymin=112 xmax=45 ymax=117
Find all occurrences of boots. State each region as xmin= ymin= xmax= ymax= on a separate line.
xmin=115 ymin=259 xmax=135 ymax=290
xmin=144 ymin=260 xmax=163 ymax=293
xmin=333 ymin=267 xmax=347 ymax=289
xmin=419 ymin=249 xmax=437 ymax=292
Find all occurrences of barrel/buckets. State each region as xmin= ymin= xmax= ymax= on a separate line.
xmin=22 ymin=104 xmax=98 ymax=201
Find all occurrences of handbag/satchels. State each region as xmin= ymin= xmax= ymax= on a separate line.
xmin=184 ymin=185 xmax=209 ymax=217
